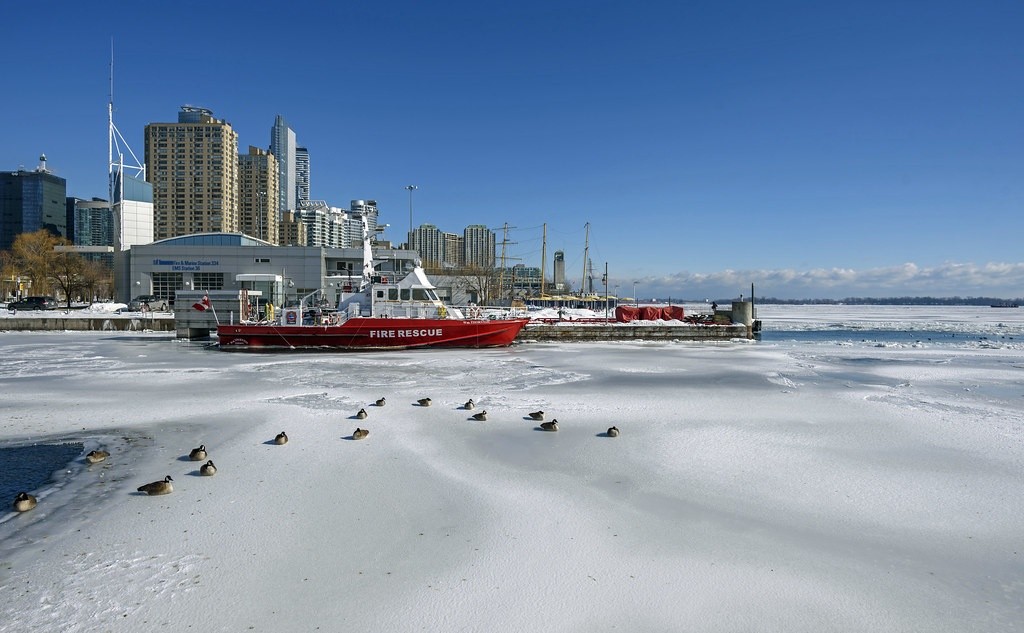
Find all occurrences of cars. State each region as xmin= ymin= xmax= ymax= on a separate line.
xmin=7 ymin=295 xmax=59 ymax=311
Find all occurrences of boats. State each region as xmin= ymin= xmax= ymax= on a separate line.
xmin=216 ymin=271 xmax=530 ymax=352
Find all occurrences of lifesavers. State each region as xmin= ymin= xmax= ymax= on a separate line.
xmin=286 ymin=311 xmax=297 ymax=320
xmin=470 ymin=306 xmax=481 ymax=317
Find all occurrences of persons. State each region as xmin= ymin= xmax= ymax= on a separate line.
xmin=467 ymin=300 xmax=478 ymax=316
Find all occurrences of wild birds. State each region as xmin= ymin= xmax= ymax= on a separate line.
xmin=86 ymin=450 xmax=111 ymax=463
xmin=136 ymin=475 xmax=174 ymax=495
xmin=188 ymin=444 xmax=207 ymax=460
xmin=540 ymin=419 xmax=560 ymax=431
xmin=274 ymin=431 xmax=288 ymax=445
xmin=465 ymin=398 xmax=474 ymax=410
xmin=353 ymin=428 xmax=369 ymax=440
xmin=199 ymin=460 xmax=217 ymax=476
xmin=14 ymin=492 xmax=37 ymax=511
xmin=528 ymin=410 xmax=544 ymax=420
xmin=472 ymin=410 xmax=487 ymax=421
xmin=356 ymin=409 xmax=368 ymax=419
xmin=606 ymin=425 xmax=620 ymax=437
xmin=376 ymin=396 xmax=386 ymax=406
xmin=417 ymin=398 xmax=432 ymax=406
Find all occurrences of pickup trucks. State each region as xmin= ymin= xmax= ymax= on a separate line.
xmin=127 ymin=295 xmax=169 ymax=313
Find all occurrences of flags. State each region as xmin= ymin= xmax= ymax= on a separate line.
xmin=192 ymin=293 xmax=213 ymax=311
